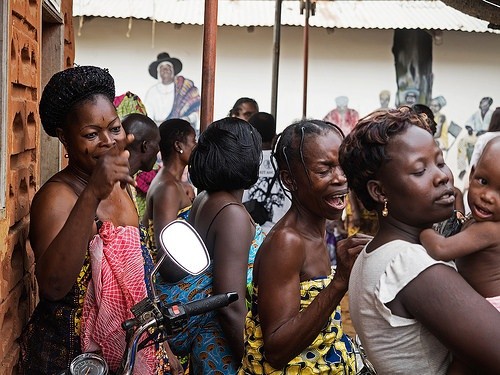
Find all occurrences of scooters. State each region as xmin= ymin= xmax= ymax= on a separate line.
xmin=59 ymin=220 xmax=240 ymax=375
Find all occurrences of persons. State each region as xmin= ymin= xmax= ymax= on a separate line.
xmin=153 ymin=118 xmax=263 ymax=375
xmin=420 ymin=134 xmax=500 ymax=375
xmin=29 ymin=65 xmax=185 ymax=375
xmin=121 ymin=97 xmax=292 ymax=251
xmin=338 ymin=104 xmax=500 ymax=375
xmin=237 ymin=121 xmax=373 ymax=375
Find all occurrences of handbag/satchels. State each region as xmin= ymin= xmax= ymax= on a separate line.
xmin=241 ymin=199 xmax=272 ymax=226
